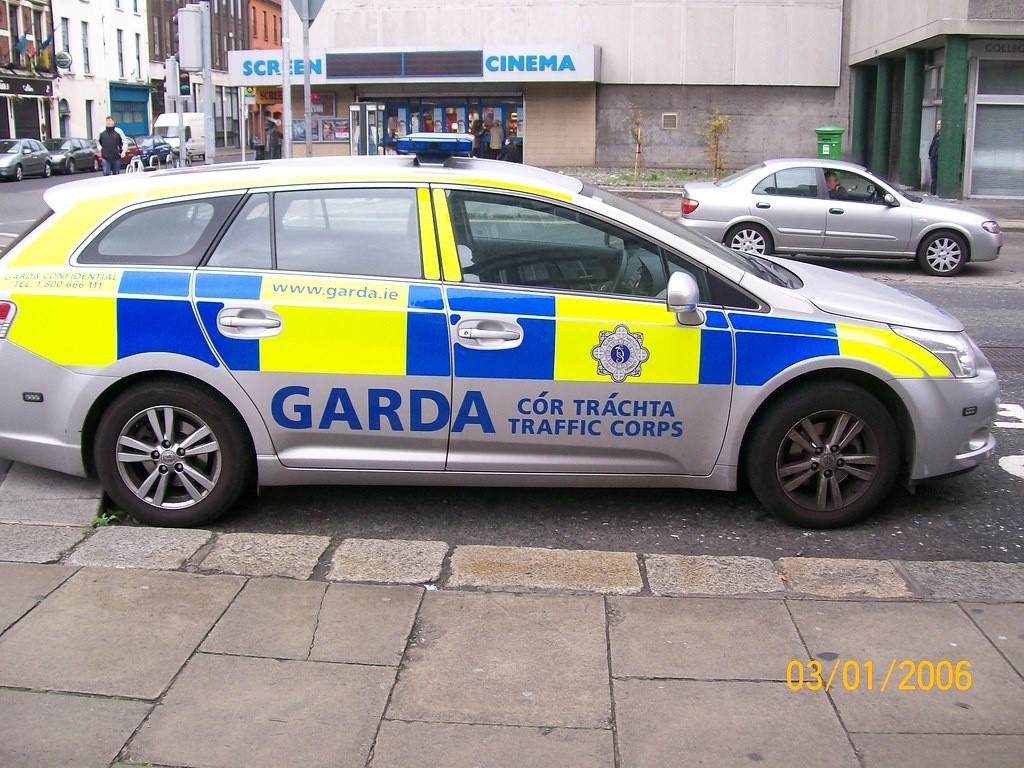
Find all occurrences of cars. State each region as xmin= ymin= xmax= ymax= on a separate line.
xmin=88 ymin=136 xmax=143 ymax=170
xmin=680 ymin=158 xmax=1003 ymax=276
xmin=42 ymin=137 xmax=100 ymax=175
xmin=0 ymin=130 xmax=1000 ymax=529
xmin=0 ymin=137 xmax=53 ymax=181
xmin=135 ymin=135 xmax=174 ymax=166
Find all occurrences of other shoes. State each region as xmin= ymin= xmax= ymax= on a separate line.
xmin=927 ymin=192 xmax=934 ymax=195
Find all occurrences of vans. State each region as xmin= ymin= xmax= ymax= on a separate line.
xmin=155 ymin=115 xmax=205 ymax=163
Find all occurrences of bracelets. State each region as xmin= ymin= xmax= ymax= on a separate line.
xmin=835 ymin=185 xmax=838 ymax=189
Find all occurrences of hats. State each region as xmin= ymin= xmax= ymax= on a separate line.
xmin=492 ymin=120 xmax=498 ymax=123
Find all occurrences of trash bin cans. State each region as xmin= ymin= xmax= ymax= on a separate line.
xmin=254 ymin=144 xmax=264 ymax=160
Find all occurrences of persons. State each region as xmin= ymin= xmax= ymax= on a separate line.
xmin=324 ymin=122 xmax=334 ymax=139
xmin=489 ymin=120 xmax=505 ymax=159
xmin=435 ymin=120 xmax=442 ymax=132
xmin=497 ymin=137 xmax=518 ymax=162
xmin=457 ymin=120 xmax=465 ymax=133
xmin=824 ymin=172 xmax=849 ymax=199
xmin=98 ymin=116 xmax=128 ymax=176
xmin=398 ymin=121 xmax=406 ymax=132
xmin=385 ymin=145 xmax=397 ymax=155
xmin=471 ymin=120 xmax=483 ymax=157
xmin=272 ymin=111 xmax=283 ymax=144
xmin=923 ymin=119 xmax=942 ymax=197
